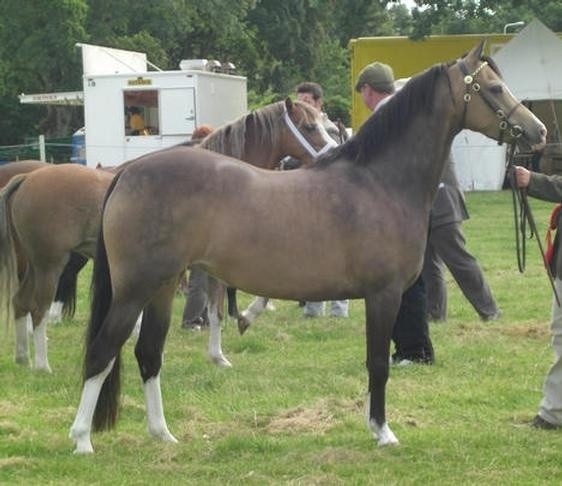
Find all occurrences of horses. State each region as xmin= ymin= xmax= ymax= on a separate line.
xmin=1 ymin=114 xmax=346 ymax=323
xmin=1 ymin=98 xmax=337 ymax=375
xmin=69 ymin=41 xmax=547 ymax=458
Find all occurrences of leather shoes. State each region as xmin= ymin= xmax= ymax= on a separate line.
xmin=482 ymin=310 xmax=502 ymax=322
xmin=513 ymin=414 xmax=561 ymax=431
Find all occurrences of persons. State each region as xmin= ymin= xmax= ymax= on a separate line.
xmin=181 ymin=127 xmax=213 ymax=330
xmin=128 ymin=106 xmax=150 ymax=136
xmin=355 ymin=62 xmax=502 ymax=325
xmin=514 ymin=166 xmax=562 ymax=430
xmin=296 ymin=83 xmax=350 ymax=320
xmin=388 ymin=273 xmax=435 ymax=366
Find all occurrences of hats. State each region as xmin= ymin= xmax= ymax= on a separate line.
xmin=355 ymin=61 xmax=396 ymax=96
